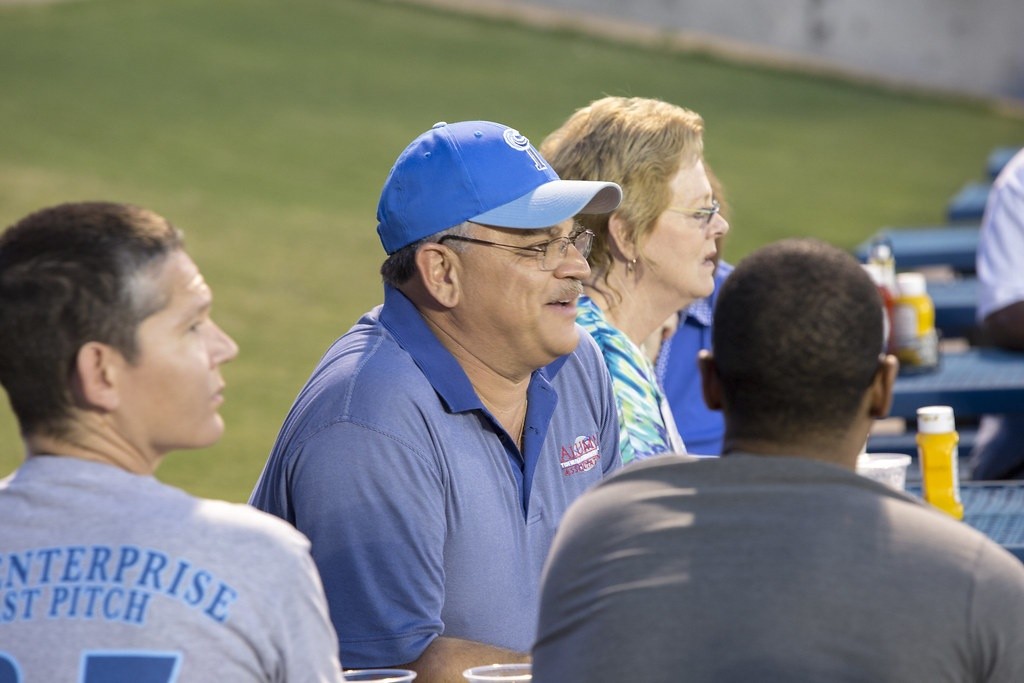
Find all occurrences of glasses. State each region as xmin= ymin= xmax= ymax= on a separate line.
xmin=659 ymin=200 xmax=722 ymax=227
xmin=437 ymin=229 xmax=595 ymax=272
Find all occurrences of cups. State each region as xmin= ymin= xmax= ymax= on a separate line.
xmin=343 ymin=669 xmax=417 ymax=683
xmin=854 ymin=453 xmax=912 ymax=493
xmin=462 ymin=664 xmax=532 ymax=683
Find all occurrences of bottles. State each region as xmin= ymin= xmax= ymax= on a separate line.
xmin=855 ymin=239 xmax=939 ymax=375
xmin=914 ymin=404 xmax=964 ymax=522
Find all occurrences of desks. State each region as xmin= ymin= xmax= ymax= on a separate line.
xmin=855 ymin=148 xmax=1024 ymax=564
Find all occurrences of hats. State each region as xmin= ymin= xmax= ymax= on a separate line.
xmin=377 ymin=119 xmax=623 ymax=255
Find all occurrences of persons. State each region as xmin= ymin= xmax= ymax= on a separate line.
xmin=538 ymin=96 xmax=736 ymax=473
xmin=531 ymin=241 xmax=1024 ymax=683
xmin=0 ymin=201 xmax=347 ymax=683
xmin=249 ymin=121 xmax=621 ymax=683
xmin=976 ymin=148 xmax=1024 ymax=355
